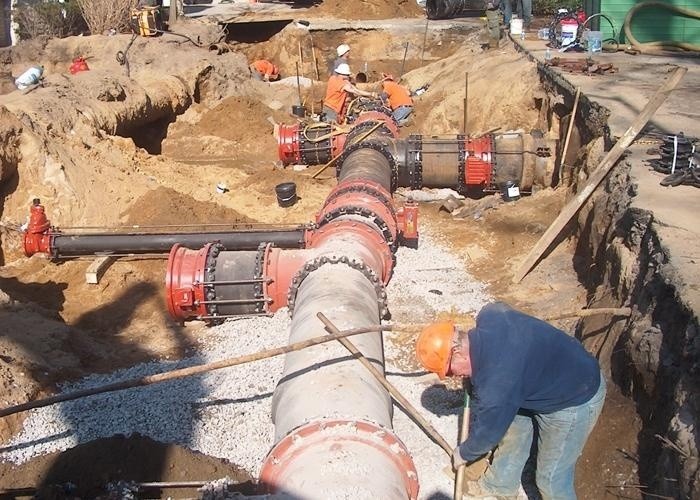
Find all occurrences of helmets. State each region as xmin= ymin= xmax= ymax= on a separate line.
xmin=415 ymin=321 xmax=454 ymax=380
xmin=336 ymin=45 xmax=350 ymax=56
xmin=334 ymin=64 xmax=352 ymax=74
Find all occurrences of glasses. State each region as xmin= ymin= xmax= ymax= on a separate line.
xmin=446 ymin=356 xmax=453 ymax=376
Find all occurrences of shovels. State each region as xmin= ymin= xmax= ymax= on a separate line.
xmin=553 ymin=87 xmax=582 ymax=190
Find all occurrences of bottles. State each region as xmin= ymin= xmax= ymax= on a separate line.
xmin=521 ymin=30 xmax=525 ymax=41
xmin=545 ymin=47 xmax=551 ymax=60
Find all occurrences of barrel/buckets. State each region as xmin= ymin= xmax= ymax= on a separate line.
xmin=583 ymin=31 xmax=589 ymax=52
xmin=274 ymin=182 xmax=297 ymax=208
xmin=510 ymin=19 xmax=523 ymax=34
xmin=297 ymin=106 xmax=307 ymax=116
xmin=292 ymin=106 xmax=296 ymax=114
xmin=586 ymin=31 xmax=602 ymax=54
xmin=498 ymin=180 xmax=520 ymax=202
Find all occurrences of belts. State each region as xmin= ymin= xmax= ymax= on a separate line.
xmin=399 ymin=105 xmax=412 ymax=107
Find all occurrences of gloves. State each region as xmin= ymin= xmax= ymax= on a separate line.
xmin=453 ymin=447 xmax=467 ymax=470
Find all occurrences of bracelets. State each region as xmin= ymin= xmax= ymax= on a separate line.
xmin=371 ymin=91 xmax=376 ymax=97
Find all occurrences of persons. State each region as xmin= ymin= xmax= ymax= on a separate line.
xmin=322 ymin=63 xmax=379 ymax=126
xmin=379 ymin=73 xmax=415 ymax=123
xmin=500 ymin=0 xmax=514 ymax=29
xmin=415 ymin=300 xmax=606 ymax=500
xmin=332 ymin=43 xmax=355 ymax=85
xmin=516 ymin=0 xmax=532 ymax=32
xmin=249 ymin=59 xmax=279 ymax=82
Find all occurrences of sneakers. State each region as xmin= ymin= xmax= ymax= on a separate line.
xmin=467 ymin=481 xmax=518 ymax=497
